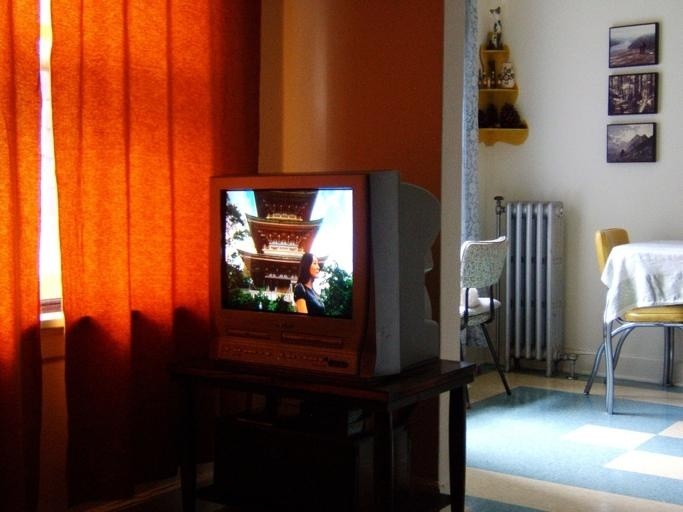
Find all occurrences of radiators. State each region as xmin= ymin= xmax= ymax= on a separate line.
xmin=492 ymin=195 xmax=580 ymax=374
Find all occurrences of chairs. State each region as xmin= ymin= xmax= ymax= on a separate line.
xmin=461 ymin=236 xmax=511 ymax=410
xmin=583 ymin=229 xmax=683 ymax=395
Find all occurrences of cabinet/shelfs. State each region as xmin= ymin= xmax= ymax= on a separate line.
xmin=478 ymin=32 xmax=529 ymax=147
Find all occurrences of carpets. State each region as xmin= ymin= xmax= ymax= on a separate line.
xmin=467 ymin=386 xmax=682 ymax=506
xmin=466 ymin=495 xmax=546 ymax=512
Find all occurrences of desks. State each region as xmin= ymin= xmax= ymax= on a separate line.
xmin=170 ymin=359 xmax=476 ymax=512
xmin=596 ymin=241 xmax=683 ymax=414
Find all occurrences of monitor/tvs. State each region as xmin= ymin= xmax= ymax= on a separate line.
xmin=208 ymin=168 xmax=443 ymax=378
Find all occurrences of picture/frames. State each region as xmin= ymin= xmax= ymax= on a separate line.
xmin=607 ymin=123 xmax=656 ymax=162
xmin=608 ymin=72 xmax=659 ymax=115
xmin=608 ymin=22 xmax=658 ymax=69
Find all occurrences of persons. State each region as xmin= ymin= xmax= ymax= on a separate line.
xmin=294 ymin=254 xmax=327 ymax=318
